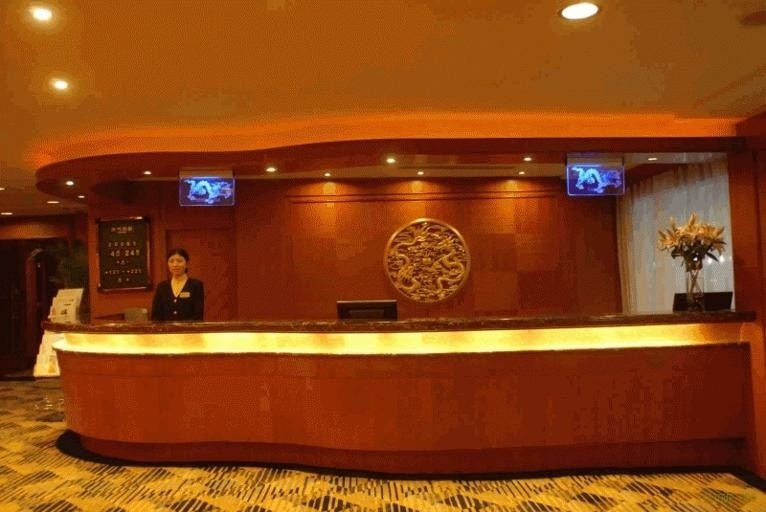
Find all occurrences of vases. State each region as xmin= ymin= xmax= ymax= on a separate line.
xmin=684 ymin=262 xmax=705 ymax=312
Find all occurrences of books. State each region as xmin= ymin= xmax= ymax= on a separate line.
xmin=33 ymin=288 xmax=84 ymax=377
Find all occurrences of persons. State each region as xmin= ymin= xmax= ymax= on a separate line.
xmin=150 ymin=248 xmax=204 ymax=322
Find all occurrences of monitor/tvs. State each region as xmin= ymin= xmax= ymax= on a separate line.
xmin=95 ymin=313 xmax=126 ymax=321
xmin=336 ymin=299 xmax=398 ymax=320
xmin=673 ymin=291 xmax=733 ymax=311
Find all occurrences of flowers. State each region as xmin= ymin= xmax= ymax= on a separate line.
xmin=653 ymin=209 xmax=733 ymax=293
